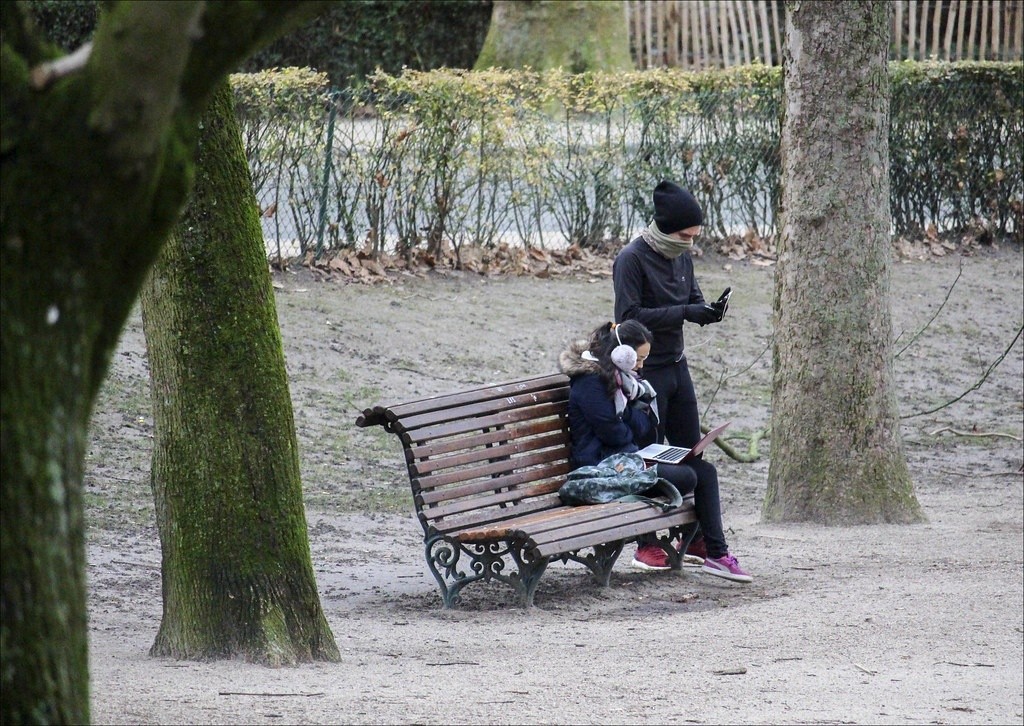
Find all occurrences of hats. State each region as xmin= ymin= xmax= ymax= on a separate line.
xmin=652 ymin=180 xmax=706 ymax=234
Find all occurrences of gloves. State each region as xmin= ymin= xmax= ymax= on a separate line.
xmin=711 ymin=286 xmax=731 ymax=321
xmin=684 ymin=304 xmax=721 ymax=327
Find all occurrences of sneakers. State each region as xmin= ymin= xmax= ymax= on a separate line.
xmin=632 ymin=541 xmax=674 ymax=571
xmin=701 ymin=552 xmax=753 ymax=582
xmin=676 ymin=534 xmax=707 ymax=564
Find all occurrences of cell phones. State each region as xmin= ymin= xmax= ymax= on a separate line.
xmin=721 ymin=291 xmax=734 ymax=302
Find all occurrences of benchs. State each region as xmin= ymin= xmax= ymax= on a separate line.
xmin=355 ymin=370 xmax=699 ymax=609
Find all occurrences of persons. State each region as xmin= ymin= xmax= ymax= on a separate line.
xmin=558 ymin=319 xmax=754 ymax=582
xmin=612 ymin=181 xmax=731 ymax=571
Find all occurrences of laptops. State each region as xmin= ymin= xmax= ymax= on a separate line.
xmin=638 ymin=422 xmax=732 ymax=463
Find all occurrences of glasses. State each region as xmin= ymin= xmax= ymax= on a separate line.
xmin=676 ymin=230 xmax=696 ymax=242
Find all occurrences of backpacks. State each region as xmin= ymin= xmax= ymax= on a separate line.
xmin=558 ymin=451 xmax=682 ymax=514
